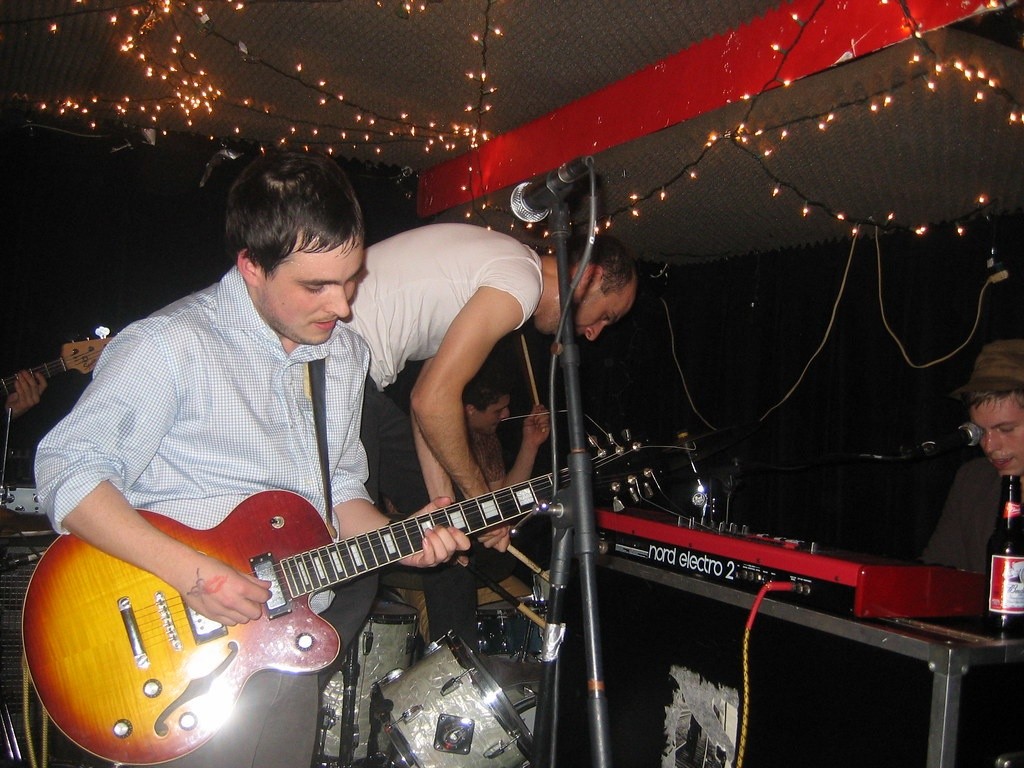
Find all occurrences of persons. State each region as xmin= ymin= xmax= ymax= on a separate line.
xmin=32 ymin=178 xmax=472 ymax=768
xmin=0 ymin=361 xmax=52 ymax=431
xmin=316 ymin=221 xmax=637 ymax=692
xmin=916 ymin=338 xmax=1024 ymax=574
xmin=384 ymin=370 xmax=555 ymax=645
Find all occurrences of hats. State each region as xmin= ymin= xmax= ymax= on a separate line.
xmin=949 ymin=338 xmax=1024 ymax=399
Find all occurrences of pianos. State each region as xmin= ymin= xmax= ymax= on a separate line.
xmin=594 ymin=503 xmax=991 ymax=623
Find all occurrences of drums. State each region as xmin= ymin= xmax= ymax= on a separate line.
xmin=475 ymin=596 xmax=553 ymax=657
xmin=370 ymin=627 xmax=534 ymax=768
xmin=314 ymin=600 xmax=420 ymax=766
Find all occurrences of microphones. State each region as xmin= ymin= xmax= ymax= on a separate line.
xmin=922 ymin=422 xmax=982 ymax=456
xmin=510 ymin=155 xmax=595 ymax=223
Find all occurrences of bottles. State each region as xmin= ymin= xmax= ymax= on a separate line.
xmin=984 ymin=476 xmax=1024 ymax=639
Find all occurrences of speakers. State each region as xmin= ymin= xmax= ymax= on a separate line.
xmin=0 ymin=505 xmax=62 ymax=768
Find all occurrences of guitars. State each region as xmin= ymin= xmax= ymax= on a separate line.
xmin=20 ymin=427 xmax=677 ymax=767
xmin=0 ymin=326 xmax=114 ymax=407
